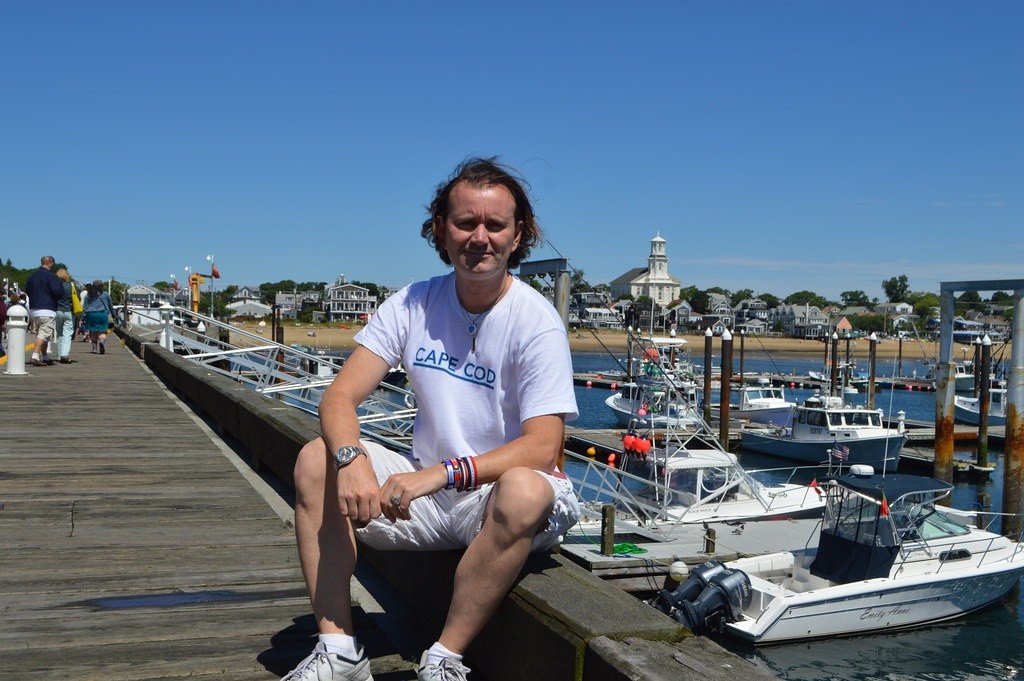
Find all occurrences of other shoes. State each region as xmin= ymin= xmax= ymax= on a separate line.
xmin=83 ymin=332 xmax=88 ymax=342
xmin=30 ymin=358 xmax=47 ymax=367
xmin=42 ymin=358 xmax=55 ymax=366
xmin=56 ymin=357 xmax=73 ymax=364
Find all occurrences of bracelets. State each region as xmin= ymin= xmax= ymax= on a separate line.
xmin=441 ymin=456 xmax=478 ymax=493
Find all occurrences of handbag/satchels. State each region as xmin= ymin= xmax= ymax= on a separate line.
xmin=108 ymin=311 xmax=114 ymax=323
xmin=72 ymin=283 xmax=83 ymax=316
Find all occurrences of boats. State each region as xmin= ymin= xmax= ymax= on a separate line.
xmin=559 ymin=319 xmax=1024 ymax=647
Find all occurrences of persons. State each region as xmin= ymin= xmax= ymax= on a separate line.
xmin=277 ymin=155 xmax=581 ymax=681
xmin=25 ymin=256 xmax=116 ymax=365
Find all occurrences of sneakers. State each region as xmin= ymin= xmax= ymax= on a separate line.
xmin=417 ymin=650 xmax=471 ymax=681
xmin=276 ymin=641 xmax=375 ymax=681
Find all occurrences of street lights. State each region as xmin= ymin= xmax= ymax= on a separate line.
xmin=169 ymin=273 xmax=176 ymax=305
xmin=3 ymin=277 xmax=9 ymax=300
xmin=206 ymin=254 xmax=216 ymax=319
xmin=184 ymin=266 xmax=191 ymax=310
xmin=13 ymin=282 xmax=18 ymax=293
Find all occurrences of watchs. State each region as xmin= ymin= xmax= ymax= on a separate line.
xmin=334 ymin=446 xmax=367 ymax=473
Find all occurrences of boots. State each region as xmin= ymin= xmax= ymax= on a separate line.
xmin=91 ymin=340 xmax=98 ymax=353
xmin=99 ymin=336 xmax=105 ymax=354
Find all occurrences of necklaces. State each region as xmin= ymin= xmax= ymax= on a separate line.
xmin=453 ymin=276 xmax=507 ymax=336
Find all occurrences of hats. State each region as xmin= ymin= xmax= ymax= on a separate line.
xmin=93 ymin=280 xmax=102 ymax=285
